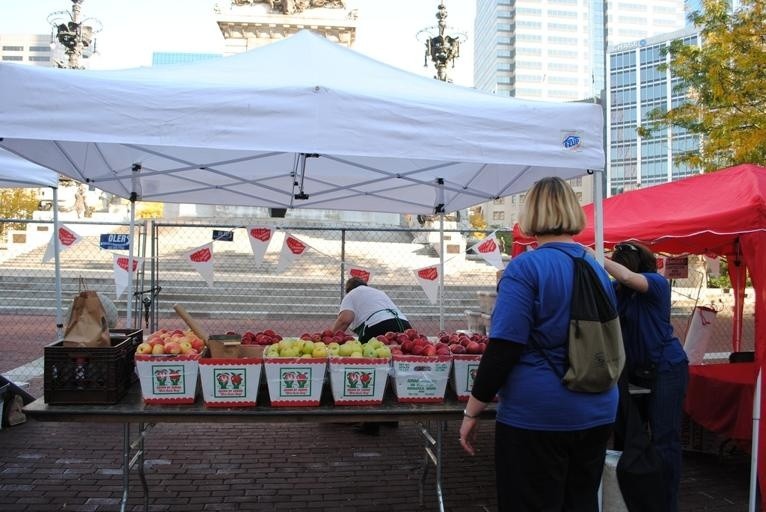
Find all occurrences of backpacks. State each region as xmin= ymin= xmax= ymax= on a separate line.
xmin=529 ymin=246 xmax=626 ymax=392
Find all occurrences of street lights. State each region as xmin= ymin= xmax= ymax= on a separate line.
xmin=415 ymin=0 xmax=468 ymax=86
xmin=46 ymin=1 xmax=104 ymax=70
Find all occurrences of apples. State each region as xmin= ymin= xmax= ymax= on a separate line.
xmin=216 ymin=372 xmax=242 ymax=384
xmin=155 ymin=369 xmax=181 ymax=381
xmin=225 ymin=329 xmax=489 ymax=359
xmin=347 ymin=371 xmax=373 ymax=383
xmin=137 ymin=327 xmax=204 ymax=356
xmin=283 ymin=371 xmax=308 ymax=382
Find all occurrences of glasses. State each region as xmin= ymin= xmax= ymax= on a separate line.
xmin=615 ymin=244 xmax=638 ymax=251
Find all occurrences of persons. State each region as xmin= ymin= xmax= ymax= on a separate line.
xmin=332 ymin=277 xmax=412 ymax=436
xmin=576 ymin=240 xmax=689 ymax=512
xmin=459 ymin=176 xmax=626 ymax=512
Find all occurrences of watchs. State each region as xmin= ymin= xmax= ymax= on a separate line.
xmin=463 ymin=410 xmax=477 ymax=419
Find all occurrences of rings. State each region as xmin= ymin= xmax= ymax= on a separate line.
xmin=459 ymin=437 xmax=461 ymax=440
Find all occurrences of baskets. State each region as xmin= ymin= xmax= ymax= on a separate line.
xmin=44 ymin=328 xmax=143 ymax=406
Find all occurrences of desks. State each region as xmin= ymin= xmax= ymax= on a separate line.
xmin=19 ymin=373 xmax=656 ymax=512
xmin=687 ymin=361 xmax=762 ymax=489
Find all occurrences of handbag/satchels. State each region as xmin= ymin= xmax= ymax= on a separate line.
xmin=626 ymin=358 xmax=658 ymax=389
xmin=64 ymin=274 xmax=111 ymax=347
